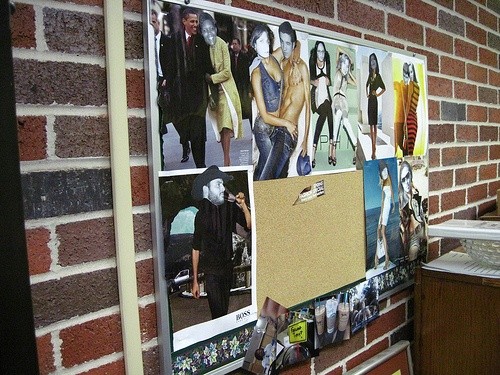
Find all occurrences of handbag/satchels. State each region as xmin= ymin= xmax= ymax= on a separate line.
xmin=377 ymin=236 xmax=386 ymax=260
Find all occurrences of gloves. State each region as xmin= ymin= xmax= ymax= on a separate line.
xmin=209 ymin=94 xmax=217 ymax=111
xmin=163 ymin=88 xmax=170 ymax=104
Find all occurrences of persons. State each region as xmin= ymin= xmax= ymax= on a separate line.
xmin=191 ymin=164 xmax=250 ymax=320
xmin=259 ymin=22 xmax=310 ymax=181
xmin=404 ymin=65 xmax=419 ymax=156
xmin=365 ymin=54 xmax=385 ymax=160
xmin=395 ymin=63 xmax=409 ymax=158
xmin=229 ymin=39 xmax=251 ymax=129
xmin=311 ymin=41 xmax=334 ymax=169
xmin=332 ymin=47 xmax=357 ymax=167
xmin=200 ymin=14 xmax=244 ymax=167
xmin=171 ymin=7 xmax=220 ymax=169
xmin=255 ymin=343 xmax=273 ymax=367
xmin=151 ymin=10 xmax=174 ymax=171
xmin=399 ymin=161 xmax=426 ymax=263
xmin=250 ymin=25 xmax=302 ymax=179
xmin=373 ymin=168 xmax=396 ymax=270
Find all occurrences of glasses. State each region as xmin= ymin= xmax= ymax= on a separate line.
xmin=401 ymin=172 xmax=412 ymax=183
xmin=152 ymin=20 xmax=158 ymax=23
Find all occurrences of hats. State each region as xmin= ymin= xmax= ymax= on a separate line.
xmin=190 ymin=166 xmax=234 ymax=202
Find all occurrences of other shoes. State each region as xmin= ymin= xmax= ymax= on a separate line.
xmin=383 ymin=262 xmax=391 ymax=270
xmin=312 ymin=159 xmax=316 ymax=168
xmin=332 ymin=158 xmax=336 ymax=166
xmin=328 ymin=156 xmax=332 ymax=165
xmin=374 ymin=263 xmax=379 ymax=270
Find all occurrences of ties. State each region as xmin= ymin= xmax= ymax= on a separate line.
xmin=187 ymin=37 xmax=192 ymax=52
